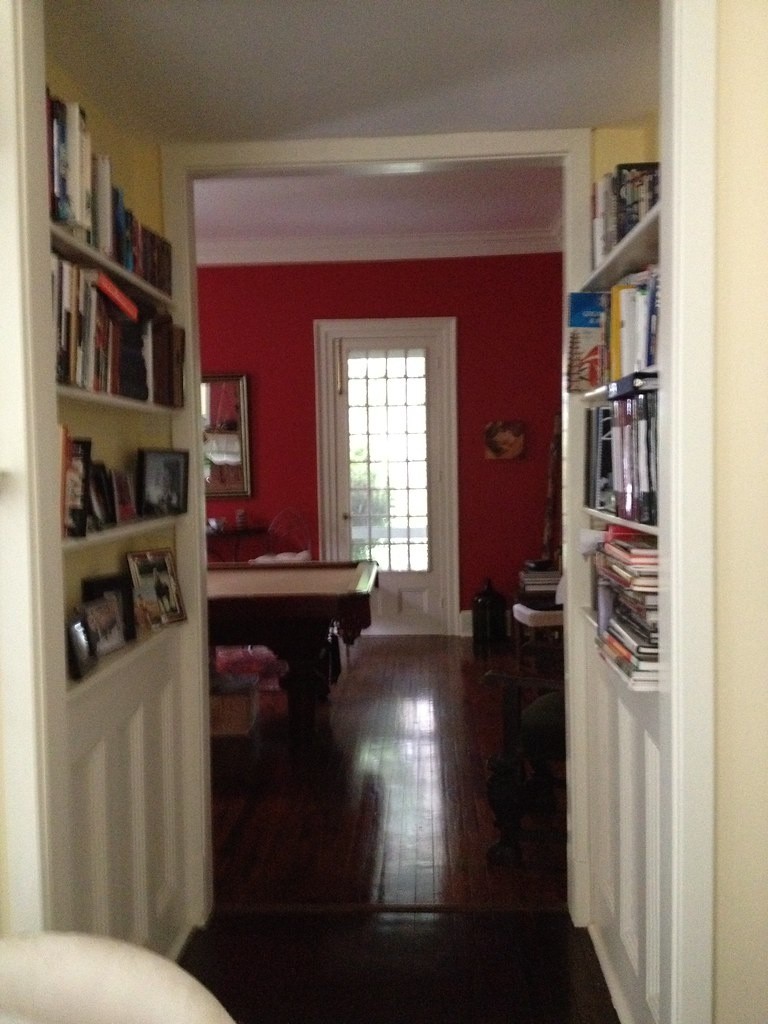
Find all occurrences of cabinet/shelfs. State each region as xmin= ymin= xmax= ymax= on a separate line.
xmin=50 ymin=223 xmax=187 ymax=699
xmin=580 ymin=198 xmax=659 ymax=739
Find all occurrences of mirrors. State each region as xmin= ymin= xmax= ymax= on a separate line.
xmin=200 ymin=372 xmax=254 ymax=499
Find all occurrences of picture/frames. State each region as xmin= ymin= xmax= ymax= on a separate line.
xmin=124 ymin=544 xmax=187 ymax=625
xmin=82 ymin=570 xmax=138 ymax=655
xmin=135 ymin=448 xmax=188 ymax=514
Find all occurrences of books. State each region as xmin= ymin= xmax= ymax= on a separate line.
xmin=596 ymin=525 xmax=660 ymax=683
xmin=568 ymin=265 xmax=660 ymax=392
xmin=584 ymin=391 xmax=657 ymax=527
xmin=47 ymin=83 xmax=173 ymax=296
xmin=590 ymin=161 xmax=660 ymax=269
xmin=518 ymin=570 xmax=561 ymax=591
xmin=49 ymin=254 xmax=188 ymax=408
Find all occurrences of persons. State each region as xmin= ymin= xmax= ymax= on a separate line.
xmin=164 ymin=556 xmax=182 ymax=613
xmin=159 ymin=474 xmax=178 ymax=506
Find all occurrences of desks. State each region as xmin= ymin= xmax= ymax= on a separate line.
xmin=208 ymin=558 xmax=379 ymax=769
xmin=207 ymin=432 xmax=242 ymax=483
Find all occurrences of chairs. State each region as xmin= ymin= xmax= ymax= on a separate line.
xmin=486 ymin=684 xmax=563 ymax=865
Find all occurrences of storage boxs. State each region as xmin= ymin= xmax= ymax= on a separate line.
xmin=208 ymin=689 xmax=257 ymax=735
